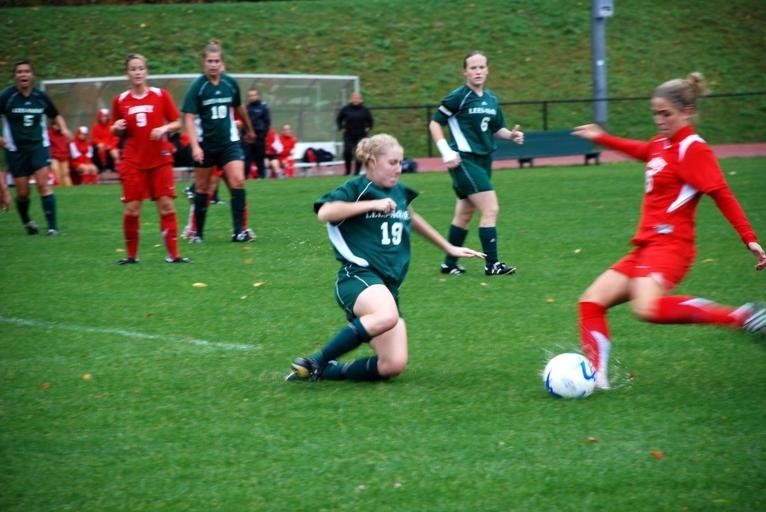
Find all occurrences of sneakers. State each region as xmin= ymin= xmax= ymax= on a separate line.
xmin=230 ymin=228 xmax=256 ymax=243
xmin=744 ymin=308 xmax=766 ymax=337
xmin=484 ymin=263 xmax=517 ymax=276
xmin=164 ymin=256 xmax=191 ymax=263
xmin=22 ymin=220 xmax=39 ymax=235
xmin=439 ymin=263 xmax=466 ymax=276
xmin=47 ymin=228 xmax=59 ymax=237
xmin=594 ymin=369 xmax=612 ymax=392
xmin=285 ymin=359 xmax=337 ymax=381
xmin=116 ymin=256 xmax=139 ymax=265
xmin=183 ymin=231 xmax=203 ymax=243
xmin=291 ymin=357 xmax=322 ymax=383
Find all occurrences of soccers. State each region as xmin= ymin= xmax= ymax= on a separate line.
xmin=542 ymin=353 xmax=595 ymax=398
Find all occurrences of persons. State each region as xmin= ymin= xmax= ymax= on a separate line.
xmin=47 ymin=121 xmax=73 ymax=186
xmin=0 ymin=137 xmax=11 ymax=212
xmin=428 ymin=53 xmax=523 ymax=276
xmin=264 ymin=129 xmax=284 ymax=178
xmin=286 ymin=134 xmax=486 ymax=384
xmin=90 ymin=109 xmax=120 ymax=174
xmin=110 ymin=53 xmax=192 ymax=265
xmin=69 ymin=126 xmax=99 ymax=184
xmin=181 ymin=110 xmax=257 ymax=241
xmin=181 ymin=39 xmax=257 ymax=242
xmin=270 ymin=125 xmax=297 ymax=178
xmin=0 ymin=62 xmax=73 ymax=237
xmin=336 ymin=91 xmax=374 ymax=177
xmin=242 ymin=90 xmax=271 ymax=180
xmin=185 ymin=182 xmax=226 ymax=205
xmin=571 ymin=73 xmax=765 ymax=391
xmin=174 ymin=132 xmax=192 ymax=168
